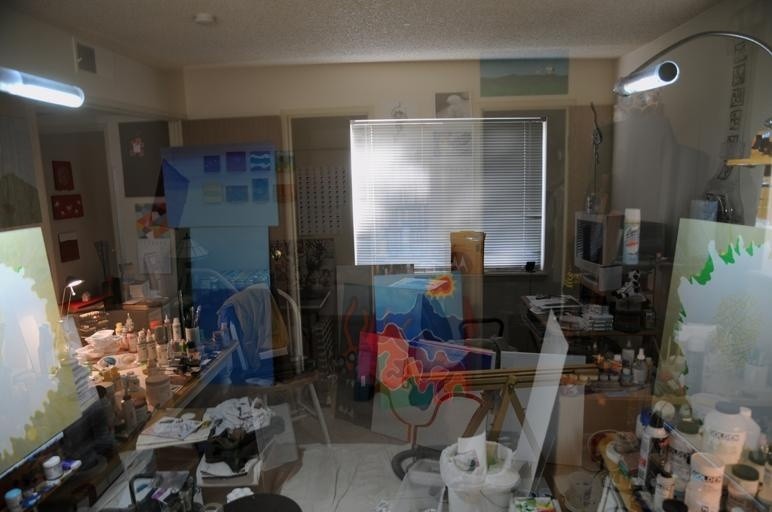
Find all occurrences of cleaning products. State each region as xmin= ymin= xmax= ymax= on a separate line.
xmin=145 ymin=367 xmax=173 ymax=407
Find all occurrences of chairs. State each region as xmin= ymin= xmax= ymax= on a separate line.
xmin=418 ymin=293 xmax=515 ymax=371
xmin=215 ymin=282 xmax=334 ymax=455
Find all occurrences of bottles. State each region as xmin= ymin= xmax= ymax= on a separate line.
xmin=593 ymin=339 xmax=655 ymax=388
xmin=89 ymin=293 xmax=231 ymax=435
xmin=4 ymin=488 xmax=23 ymax=509
xmin=622 ymin=207 xmax=642 ymax=265
xmin=639 ymin=311 xmax=657 ymax=352
xmin=636 ymin=399 xmax=771 ymax=512
xmin=42 ymin=455 xmax=62 ymax=480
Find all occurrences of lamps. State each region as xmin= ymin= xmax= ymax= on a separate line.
xmin=613 ymin=30 xmax=772 ymax=98
xmin=62 ymin=278 xmax=82 ymax=315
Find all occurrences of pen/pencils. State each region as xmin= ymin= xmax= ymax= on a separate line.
xmin=186 ymin=305 xmax=201 ymax=329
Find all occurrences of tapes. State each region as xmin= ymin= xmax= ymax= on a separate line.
xmin=605 ymin=441 xmax=622 ymax=465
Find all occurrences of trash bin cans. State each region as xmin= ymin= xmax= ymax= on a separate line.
xmin=439 ymin=440 xmax=514 ymax=512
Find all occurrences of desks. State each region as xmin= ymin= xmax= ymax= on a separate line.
xmin=53 ymin=327 xmax=244 ymax=504
xmin=523 ymin=294 xmax=663 ymax=470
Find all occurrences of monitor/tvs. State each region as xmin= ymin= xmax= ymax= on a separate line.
xmin=574 ymin=212 xmax=622 ymax=277
